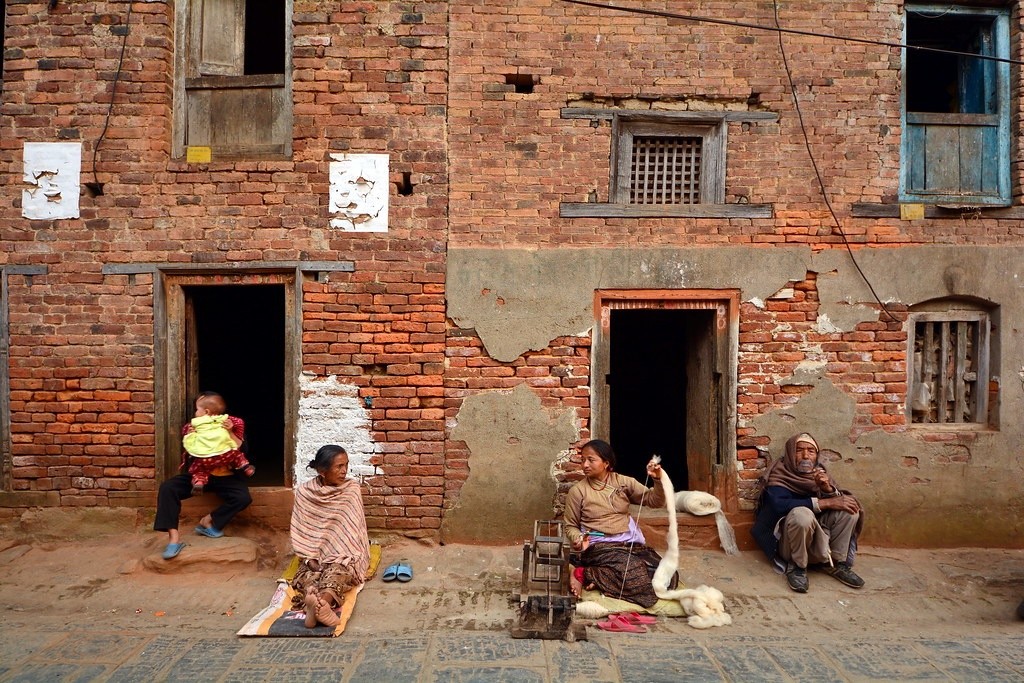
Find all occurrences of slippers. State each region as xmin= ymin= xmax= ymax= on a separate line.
xmin=381 ymin=562 xmax=400 ymax=581
xmin=608 ymin=611 xmax=657 ymax=625
xmin=194 ymin=526 xmax=224 ymax=538
xmin=397 ymin=562 xmax=413 ymax=582
xmin=162 ymin=542 xmax=186 ymax=558
xmin=597 ymin=617 xmax=647 ymax=633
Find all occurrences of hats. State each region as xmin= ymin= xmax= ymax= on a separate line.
xmin=796 ymin=434 xmax=819 ymax=452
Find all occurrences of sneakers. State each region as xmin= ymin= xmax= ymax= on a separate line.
xmin=821 ymin=559 xmax=865 ymax=588
xmin=786 ymin=559 xmax=809 ymax=593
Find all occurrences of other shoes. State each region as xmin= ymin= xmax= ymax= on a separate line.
xmin=244 ymin=464 xmax=255 ymax=476
xmin=191 ymin=481 xmax=204 ymax=498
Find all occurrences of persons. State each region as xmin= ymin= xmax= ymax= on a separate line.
xmin=152 ymin=391 xmax=254 ymax=560
xmin=290 ymin=445 xmax=370 ymax=628
xmin=750 ymin=431 xmax=866 ymax=591
xmin=563 ymin=440 xmax=679 ymax=608
xmin=181 ymin=394 xmax=255 ymax=494
